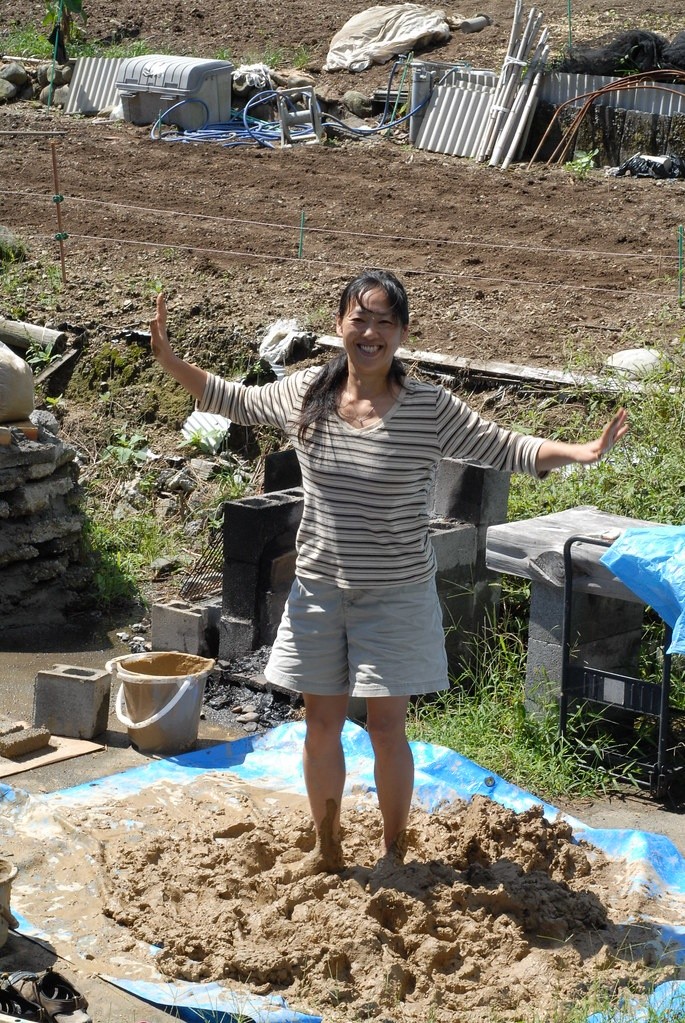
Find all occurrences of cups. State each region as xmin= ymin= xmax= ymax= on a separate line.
xmin=461 ymin=16 xmax=487 ymax=33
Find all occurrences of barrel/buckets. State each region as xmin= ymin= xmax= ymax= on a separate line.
xmin=0 ymin=858 xmax=20 ymax=949
xmin=106 ymin=652 xmax=215 ymax=753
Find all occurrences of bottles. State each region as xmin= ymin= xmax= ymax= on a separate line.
xmin=638 ymin=154 xmax=671 ymax=170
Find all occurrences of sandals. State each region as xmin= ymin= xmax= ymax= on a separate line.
xmin=0 ymin=966 xmax=93 ymax=1023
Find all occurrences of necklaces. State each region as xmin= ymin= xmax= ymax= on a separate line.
xmin=347 ymin=377 xmax=389 ymax=423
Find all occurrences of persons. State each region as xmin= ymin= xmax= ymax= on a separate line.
xmin=149 ymin=268 xmax=631 ymax=899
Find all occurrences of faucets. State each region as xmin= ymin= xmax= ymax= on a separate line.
xmin=396 ymin=53 xmax=408 ymax=64
xmin=463 ymin=62 xmax=473 ymax=71
